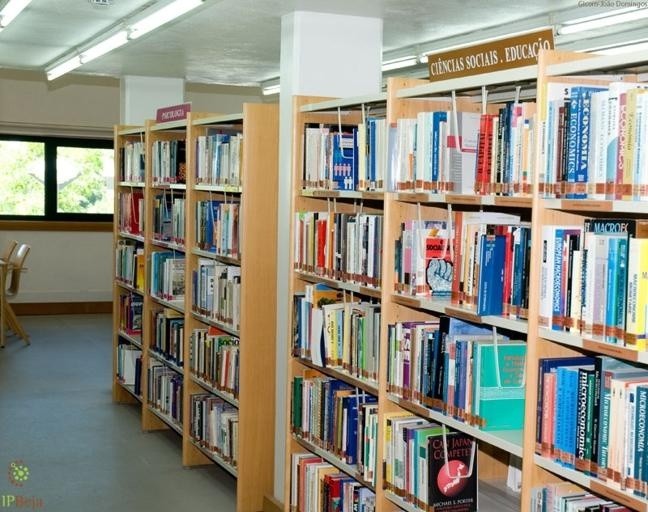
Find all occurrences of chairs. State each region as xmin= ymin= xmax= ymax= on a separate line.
xmin=0 ymin=239 xmax=31 ymax=348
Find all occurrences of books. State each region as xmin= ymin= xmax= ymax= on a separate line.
xmin=114 ymin=128 xmax=241 ymax=468
xmin=286 ymin=74 xmax=647 ymax=512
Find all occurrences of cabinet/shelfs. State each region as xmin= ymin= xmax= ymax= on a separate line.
xmin=110 ymin=102 xmax=278 ymax=512
xmin=282 ymin=48 xmax=647 ymax=512
